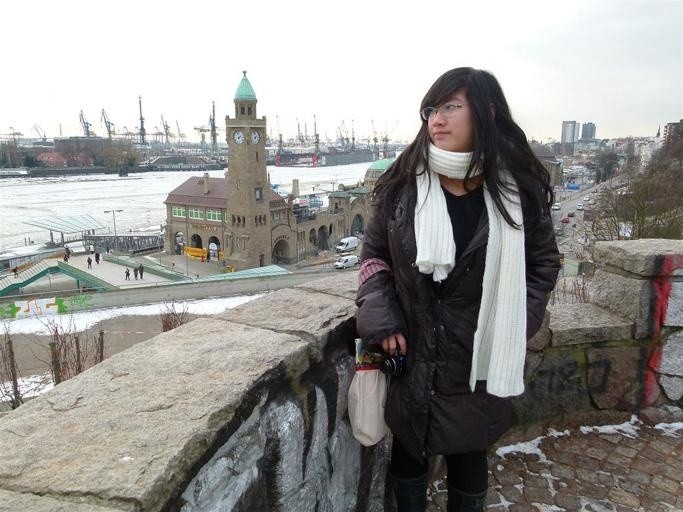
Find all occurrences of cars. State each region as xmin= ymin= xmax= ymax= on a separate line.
xmin=548 ymin=188 xmax=600 ymax=236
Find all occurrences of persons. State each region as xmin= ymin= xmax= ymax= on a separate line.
xmin=355 ymin=67 xmax=559 ymax=511
xmin=134 ymin=267 xmax=138 ymax=279
xmin=125 ymin=268 xmax=130 ymax=280
xmin=14 ymin=266 xmax=18 ymax=278
xmin=64 ymin=247 xmax=110 ymax=269
xmin=138 ymin=264 xmax=144 ymax=279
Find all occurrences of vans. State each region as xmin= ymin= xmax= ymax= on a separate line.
xmin=333 ymin=235 xmax=359 ymax=270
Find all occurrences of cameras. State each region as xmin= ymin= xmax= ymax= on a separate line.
xmin=380 ymin=343 xmax=406 ymax=377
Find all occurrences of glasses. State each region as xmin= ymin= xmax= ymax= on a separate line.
xmin=418 ymin=104 xmax=474 ymax=120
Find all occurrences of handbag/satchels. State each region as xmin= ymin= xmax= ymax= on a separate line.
xmin=348 ymin=365 xmax=388 ymax=449
